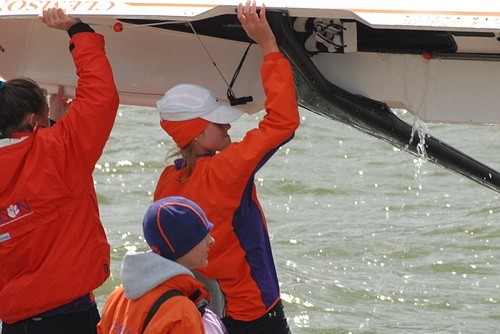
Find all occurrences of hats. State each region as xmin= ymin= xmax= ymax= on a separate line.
xmin=156 ymin=83 xmax=244 ymax=124
xmin=143 ymin=196 xmax=215 ymax=260
xmin=159 ymin=117 xmax=210 ymax=148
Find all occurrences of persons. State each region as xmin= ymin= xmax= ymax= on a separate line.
xmin=0 ymin=8 xmax=120 ymax=334
xmin=154 ymin=0 xmax=300 ymax=334
xmin=96 ymin=196 xmax=226 ymax=334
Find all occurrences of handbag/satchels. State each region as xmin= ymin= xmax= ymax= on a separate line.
xmin=141 ymin=289 xmax=228 ymax=334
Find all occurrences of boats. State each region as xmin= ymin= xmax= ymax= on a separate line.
xmin=0 ymin=0 xmax=500 ymax=195
